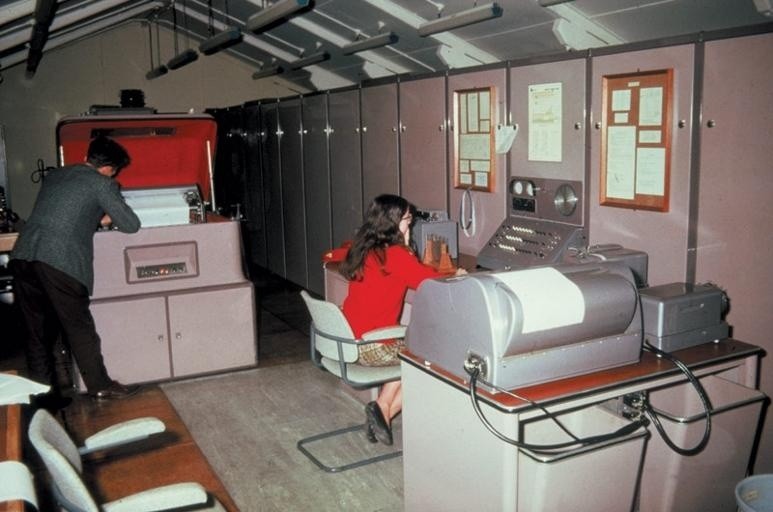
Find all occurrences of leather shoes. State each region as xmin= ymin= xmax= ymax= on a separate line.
xmin=91 ymin=382 xmax=141 ymax=403
xmin=51 ymin=396 xmax=72 ymax=414
xmin=365 ymin=401 xmax=392 ymax=445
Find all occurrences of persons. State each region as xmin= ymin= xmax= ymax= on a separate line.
xmin=341 ymin=194 xmax=468 ymax=446
xmin=7 ymin=137 xmax=141 ymax=419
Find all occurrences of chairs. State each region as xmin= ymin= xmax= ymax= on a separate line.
xmin=295 ymin=289 xmax=406 ymax=474
xmin=26 ymin=406 xmax=229 ymax=512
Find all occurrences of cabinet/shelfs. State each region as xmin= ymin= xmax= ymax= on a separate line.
xmin=75 ymin=282 xmax=257 ymax=395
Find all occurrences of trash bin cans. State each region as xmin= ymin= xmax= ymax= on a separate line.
xmin=734 ymin=473 xmax=773 ymax=512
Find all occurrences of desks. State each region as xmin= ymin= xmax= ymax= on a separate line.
xmin=322 ymin=253 xmax=769 ymax=512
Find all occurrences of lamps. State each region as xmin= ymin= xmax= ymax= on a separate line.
xmin=415 ymin=0 xmax=504 ymax=42
xmin=144 ymin=0 xmax=199 ymax=83
xmin=197 ymin=2 xmax=243 ymax=56
xmin=339 ymin=20 xmax=402 ymax=56
xmin=245 ymin=0 xmax=309 ymax=33
xmin=249 ymin=39 xmax=334 ymax=81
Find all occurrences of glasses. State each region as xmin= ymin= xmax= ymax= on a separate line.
xmin=402 ymin=214 xmax=412 ymax=220
